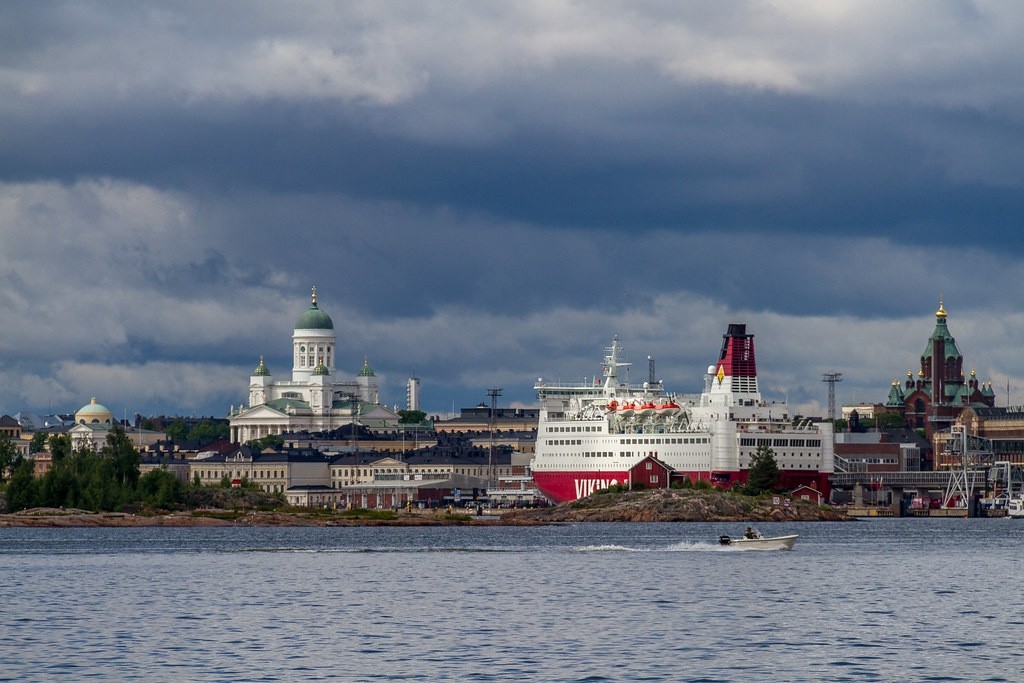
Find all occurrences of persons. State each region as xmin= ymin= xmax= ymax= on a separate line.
xmin=741 ymin=527 xmax=756 ymax=539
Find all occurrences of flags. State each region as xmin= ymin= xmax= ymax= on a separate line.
xmin=880 ymin=476 xmax=883 ymax=488
xmin=875 ymin=477 xmax=879 ymax=490
xmin=869 ymin=478 xmax=873 ymax=491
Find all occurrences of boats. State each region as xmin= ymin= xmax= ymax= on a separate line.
xmin=1007 ymin=493 xmax=1024 ymax=518
xmin=720 ymin=525 xmax=798 ymax=550
